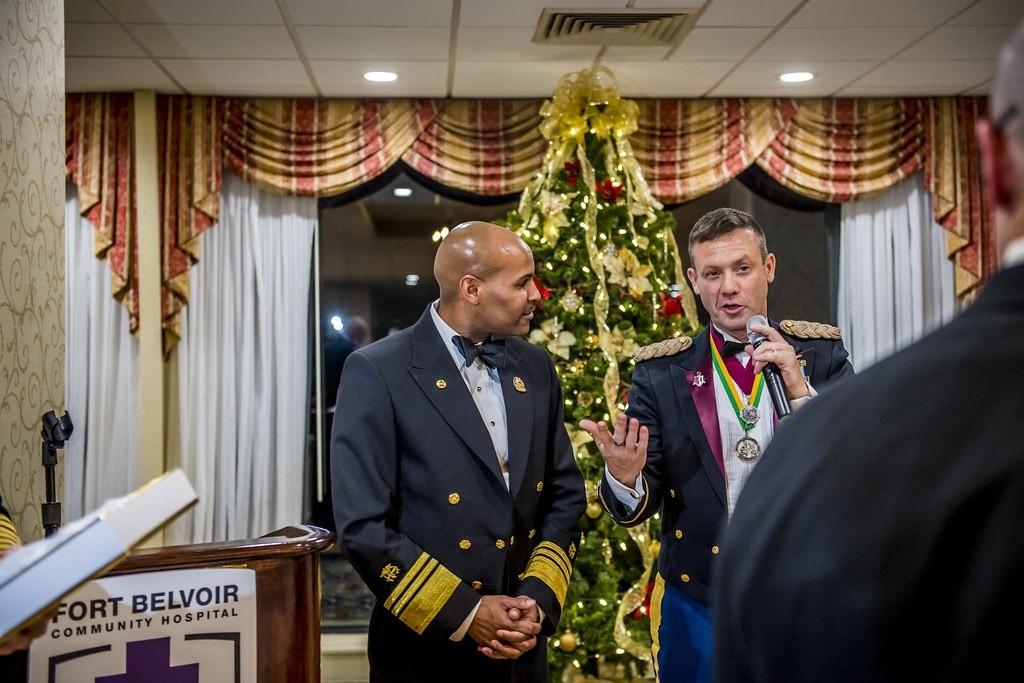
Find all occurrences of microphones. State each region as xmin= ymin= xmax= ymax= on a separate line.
xmin=747 ymin=315 xmax=793 ymax=423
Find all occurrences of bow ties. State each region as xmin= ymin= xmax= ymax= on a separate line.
xmin=452 ymin=335 xmax=506 ymax=369
xmin=712 ymin=327 xmax=753 ymax=356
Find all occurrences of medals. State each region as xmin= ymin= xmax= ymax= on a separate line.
xmin=736 ymin=436 xmax=760 ymax=463
xmin=739 ymin=405 xmax=760 ymax=425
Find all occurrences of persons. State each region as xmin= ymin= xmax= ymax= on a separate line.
xmin=329 ymin=221 xmax=587 ymax=683
xmin=578 ymin=208 xmax=855 ymax=683
xmin=330 ymin=316 xmax=368 ymax=406
xmin=708 ymin=25 xmax=1024 ymax=683
xmin=0 ymin=494 xmax=59 ymax=654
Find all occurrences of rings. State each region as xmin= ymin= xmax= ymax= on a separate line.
xmin=614 ymin=440 xmax=624 ymax=446
xmin=773 ymin=350 xmax=777 ymax=352
